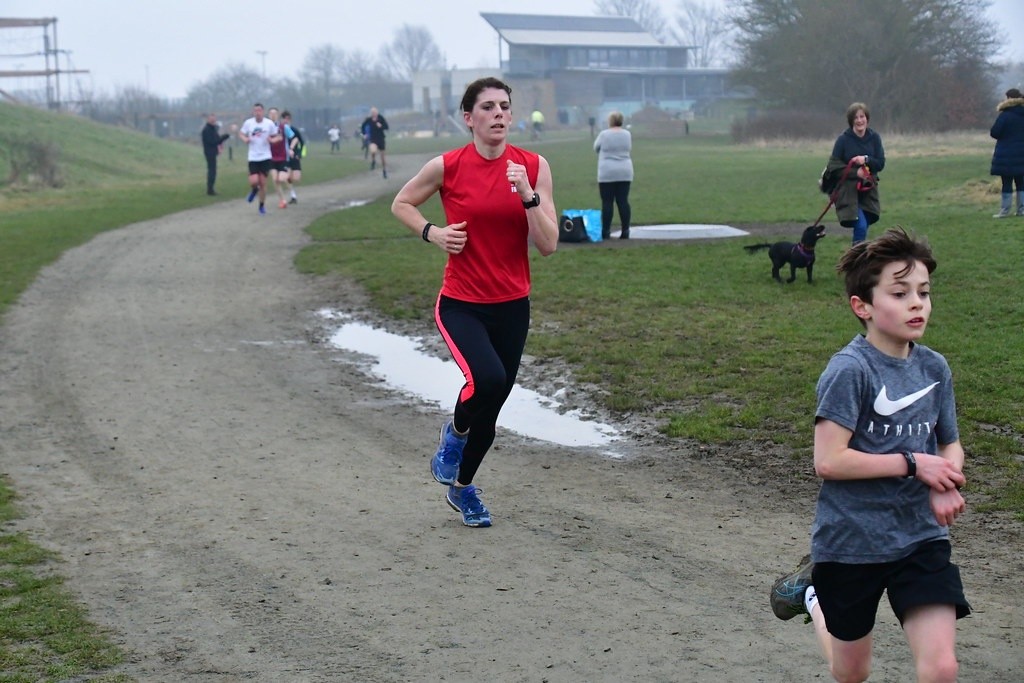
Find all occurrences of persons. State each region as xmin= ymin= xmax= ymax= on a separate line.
xmin=361 ymin=106 xmax=389 ymax=180
xmin=827 ymin=102 xmax=886 ymax=247
xmin=281 ymin=111 xmax=307 ymax=204
xmin=267 ymin=107 xmax=299 ymax=208
xmin=531 ymin=110 xmax=545 ymax=137
xmin=391 ymin=78 xmax=559 ymax=526
xmin=989 ymin=88 xmax=1024 ymax=217
xmin=356 ymin=124 xmax=368 ymax=160
xmin=239 ymin=103 xmax=283 ymax=213
xmin=770 ymin=224 xmax=974 ymax=683
xmin=202 ymin=112 xmax=230 ymax=195
xmin=328 ymin=123 xmax=340 ymax=154
xmin=593 ymin=110 xmax=633 ymax=239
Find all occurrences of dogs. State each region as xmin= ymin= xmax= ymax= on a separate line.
xmin=744 ymin=225 xmax=826 ymax=284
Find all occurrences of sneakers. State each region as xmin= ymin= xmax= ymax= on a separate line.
xmin=445 ymin=481 xmax=493 ymax=528
xmin=769 ymin=553 xmax=813 ymax=624
xmin=430 ymin=418 xmax=467 ymax=487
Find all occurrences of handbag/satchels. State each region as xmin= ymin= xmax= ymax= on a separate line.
xmin=559 ymin=215 xmax=588 ymax=243
xmin=562 ymin=209 xmax=604 ymax=244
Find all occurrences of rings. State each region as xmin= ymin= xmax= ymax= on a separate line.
xmin=512 ymin=172 xmax=515 ymax=176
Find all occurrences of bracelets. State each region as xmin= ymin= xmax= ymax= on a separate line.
xmin=290 ymin=148 xmax=294 ymax=152
xmin=422 ymin=223 xmax=436 ymax=242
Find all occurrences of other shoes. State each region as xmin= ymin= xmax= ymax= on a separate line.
xmin=382 ymin=170 xmax=387 ymax=179
xmin=603 ymin=230 xmax=611 ymax=240
xmin=619 ymin=230 xmax=630 ymax=239
xmin=287 ymin=197 xmax=297 ymax=204
xmin=258 ymin=207 xmax=265 ymax=214
xmin=207 ymin=191 xmax=218 ymax=196
xmin=369 ymin=161 xmax=376 ymax=170
xmin=278 ymin=200 xmax=287 ymax=208
xmin=247 ymin=191 xmax=256 ymax=202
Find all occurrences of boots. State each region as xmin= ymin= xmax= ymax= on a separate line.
xmin=1013 ymin=191 xmax=1024 ymax=216
xmin=992 ymin=192 xmax=1013 ymax=218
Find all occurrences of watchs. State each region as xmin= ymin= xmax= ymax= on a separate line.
xmin=522 ymin=193 xmax=540 ymax=209
xmin=898 ymin=450 xmax=916 ymax=481
